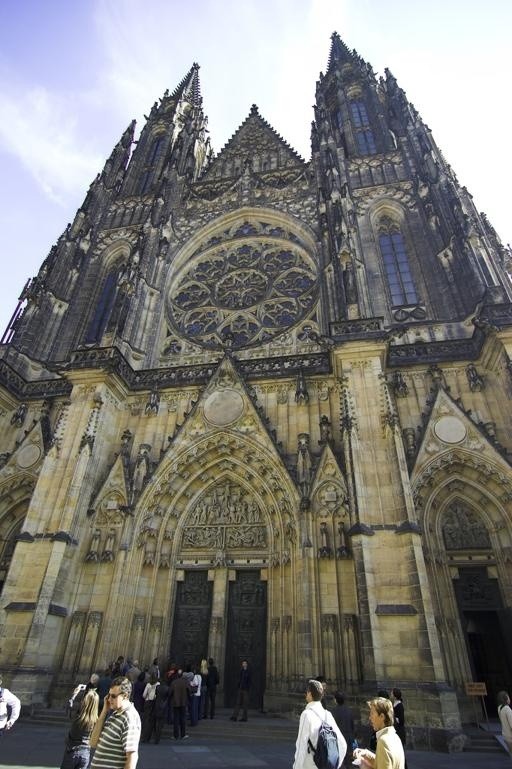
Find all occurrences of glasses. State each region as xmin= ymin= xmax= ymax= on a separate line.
xmin=108 ymin=692 xmax=125 ymax=699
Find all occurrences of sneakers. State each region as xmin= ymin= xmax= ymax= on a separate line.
xmin=139 ymin=710 xmax=249 ymax=747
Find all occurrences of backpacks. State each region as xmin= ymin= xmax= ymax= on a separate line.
xmin=305 ymin=708 xmax=340 ymax=769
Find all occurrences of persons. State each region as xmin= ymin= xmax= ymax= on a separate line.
xmin=181 ymin=483 xmax=268 ymax=548
xmin=229 ymin=660 xmax=251 ymax=722
xmin=496 ymin=691 xmax=512 ymax=756
xmin=292 ymin=676 xmax=407 ymax=769
xmin=59 ymin=657 xmax=219 ymax=769
xmin=0 ymin=674 xmax=22 ymax=736
